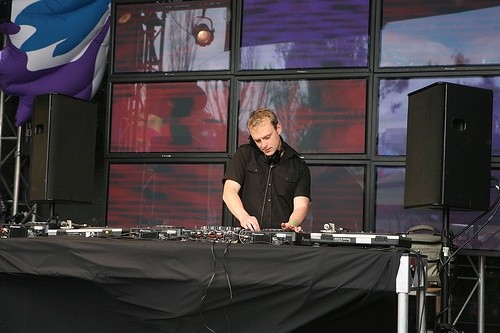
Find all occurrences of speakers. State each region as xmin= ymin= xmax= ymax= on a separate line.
xmin=28 ymin=92 xmax=98 ymax=204
xmin=402 ymin=81 xmax=493 ymax=211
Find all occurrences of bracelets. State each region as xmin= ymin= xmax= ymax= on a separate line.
xmin=289 ymin=221 xmax=297 ymax=226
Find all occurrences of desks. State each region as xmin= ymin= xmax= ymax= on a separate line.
xmin=0 ymin=239 xmax=426 ymax=333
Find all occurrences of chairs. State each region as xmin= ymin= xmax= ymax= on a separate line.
xmin=397 ymin=231 xmax=443 ymax=324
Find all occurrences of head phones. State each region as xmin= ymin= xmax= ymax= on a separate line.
xmin=247 ymin=136 xmax=285 ymax=166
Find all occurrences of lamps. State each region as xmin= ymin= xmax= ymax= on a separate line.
xmin=192 ymin=24 xmax=214 ymax=46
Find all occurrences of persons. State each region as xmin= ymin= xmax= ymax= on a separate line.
xmin=222 ymin=107 xmax=312 ymax=232
